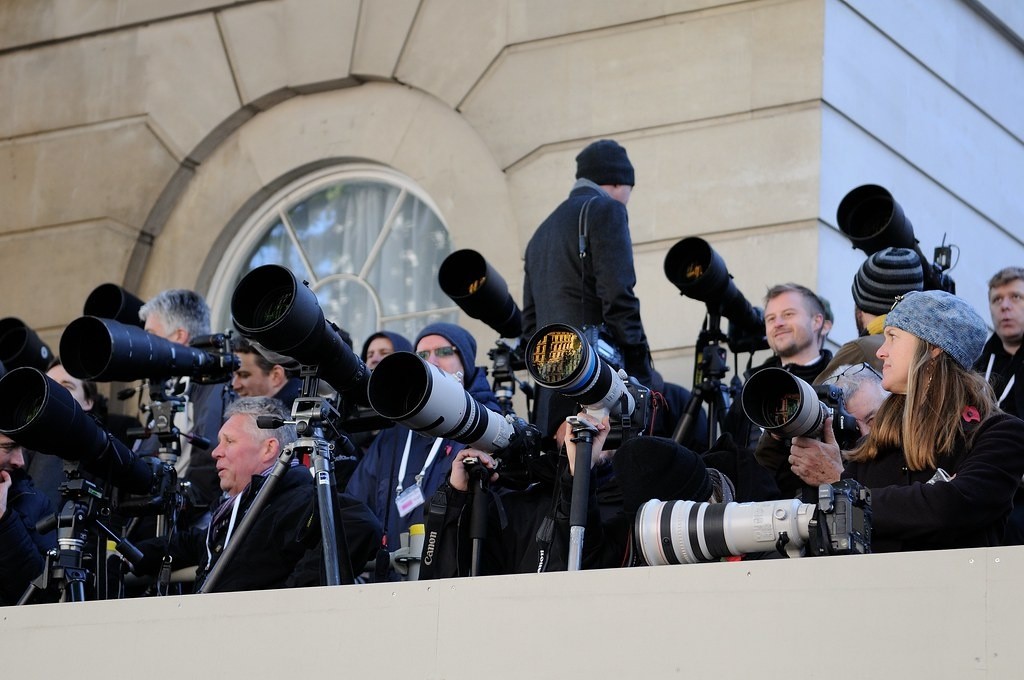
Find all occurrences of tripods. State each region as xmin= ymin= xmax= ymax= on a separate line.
xmin=673 ymin=307 xmax=728 ymax=452
xmin=196 ymin=399 xmax=356 ymax=593
xmin=16 ymin=471 xmax=144 ymax=606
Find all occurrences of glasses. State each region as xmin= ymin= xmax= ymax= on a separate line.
xmin=416 ymin=346 xmax=457 ymax=360
xmin=819 ymin=361 xmax=883 ymax=384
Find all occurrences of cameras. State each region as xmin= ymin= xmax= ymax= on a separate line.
xmin=0 ymin=367 xmax=181 ymax=515
xmin=836 ymin=185 xmax=955 ymax=297
xmin=741 ymin=368 xmax=862 ymax=451
xmin=83 ymin=283 xmax=147 ymax=331
xmin=439 ymin=250 xmax=527 ymax=370
xmin=367 ymin=352 xmax=542 ymax=481
xmin=0 ymin=317 xmax=55 ymax=373
xmin=664 ymin=237 xmax=771 ymax=354
xmin=60 ymin=317 xmax=242 ymax=386
xmin=525 ymin=323 xmax=652 ymax=429
xmin=231 ymin=264 xmax=395 ymax=434
xmin=635 ymin=478 xmax=872 ymax=566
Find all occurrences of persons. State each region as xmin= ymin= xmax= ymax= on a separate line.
xmin=0 ymin=249 xmax=1024 ymax=609
xmin=519 ymin=139 xmax=717 ymax=455
xmin=342 ymin=323 xmax=506 ymax=583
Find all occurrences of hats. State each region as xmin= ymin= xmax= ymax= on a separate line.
xmin=575 ymin=140 xmax=635 ymax=186
xmin=547 ymin=392 xmax=582 ymax=438
xmin=414 ymin=322 xmax=477 ymax=382
xmin=852 ymin=247 xmax=925 ymax=315
xmin=883 ymin=290 xmax=988 ymax=372
xmin=360 ymin=332 xmax=414 ymax=355
